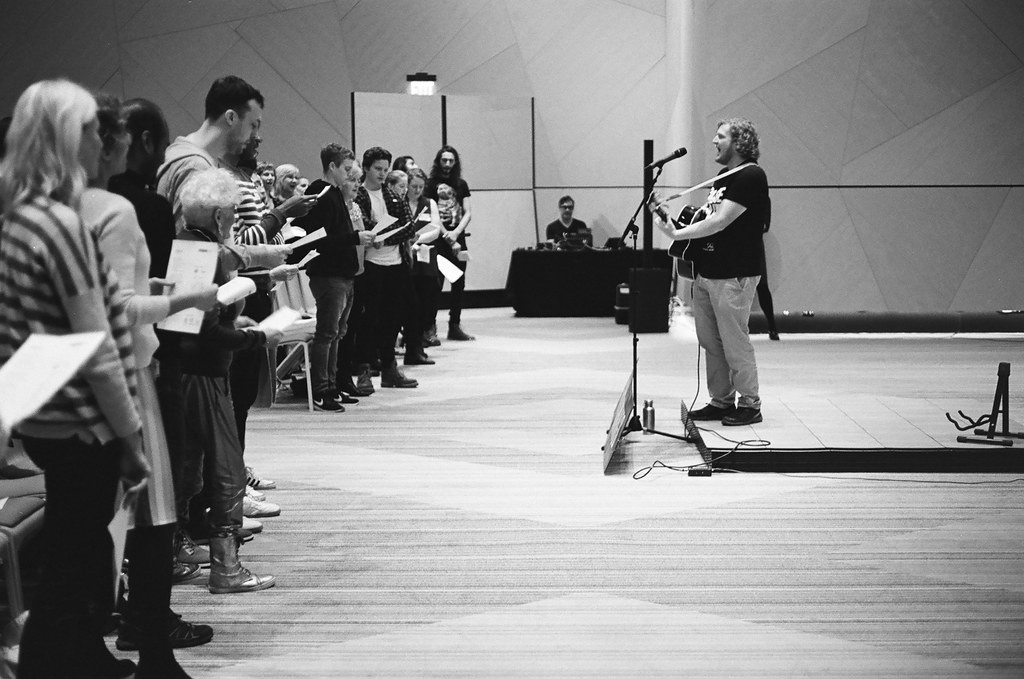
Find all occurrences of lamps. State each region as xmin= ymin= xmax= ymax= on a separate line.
xmin=406 ymin=72 xmax=437 ymax=97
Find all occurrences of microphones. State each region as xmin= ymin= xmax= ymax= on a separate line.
xmin=644 ymin=147 xmax=687 ymax=172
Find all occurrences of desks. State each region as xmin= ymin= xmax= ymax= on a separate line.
xmin=503 ymin=248 xmax=675 ymax=317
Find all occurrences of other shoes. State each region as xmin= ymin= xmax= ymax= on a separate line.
xmin=403 ymin=346 xmax=435 ymax=364
xmin=424 ymin=324 xmax=440 ymax=346
xmin=344 ymin=377 xmax=369 ymax=398
xmin=448 ymin=324 xmax=475 ymax=341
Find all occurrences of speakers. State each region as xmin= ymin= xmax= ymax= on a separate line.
xmin=629 ymin=268 xmax=668 ymax=334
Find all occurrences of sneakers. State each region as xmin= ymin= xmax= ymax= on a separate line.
xmin=308 ymin=393 xmax=345 ymax=413
xmin=721 ymin=405 xmax=764 ymax=426
xmin=168 ymin=560 xmax=201 ymax=584
xmin=171 ymin=528 xmax=212 ymax=569
xmin=685 ymin=402 xmax=736 ymax=420
xmin=331 ymin=391 xmax=360 ymax=405
xmin=114 ymin=617 xmax=213 ymax=648
xmin=244 ymin=466 xmax=277 ymax=501
xmin=241 ymin=495 xmax=281 ymax=531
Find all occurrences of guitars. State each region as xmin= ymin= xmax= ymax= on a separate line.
xmin=646 ymin=189 xmax=706 ymax=282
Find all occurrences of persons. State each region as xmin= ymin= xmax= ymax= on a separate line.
xmin=546 ymin=195 xmax=592 ymax=249
xmin=252 ymin=146 xmax=476 ymax=413
xmin=229 ymin=128 xmax=318 ymax=503
xmin=654 ymin=118 xmax=771 ymax=426
xmin=153 ymin=75 xmax=294 ymax=582
xmin=70 ymin=90 xmax=218 ymax=679
xmin=1 ymin=78 xmax=151 ymax=679
xmin=101 ymin=96 xmax=220 ymax=650
xmin=166 ymin=166 xmax=276 ymax=596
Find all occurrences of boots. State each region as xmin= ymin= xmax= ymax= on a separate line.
xmin=357 ymin=363 xmax=375 ymax=392
xmin=137 ymin=632 xmax=191 ymax=679
xmin=381 ymin=358 xmax=418 ymax=388
xmin=207 ymin=531 xmax=275 ymax=595
xmin=97 ymin=638 xmax=137 ymax=679
